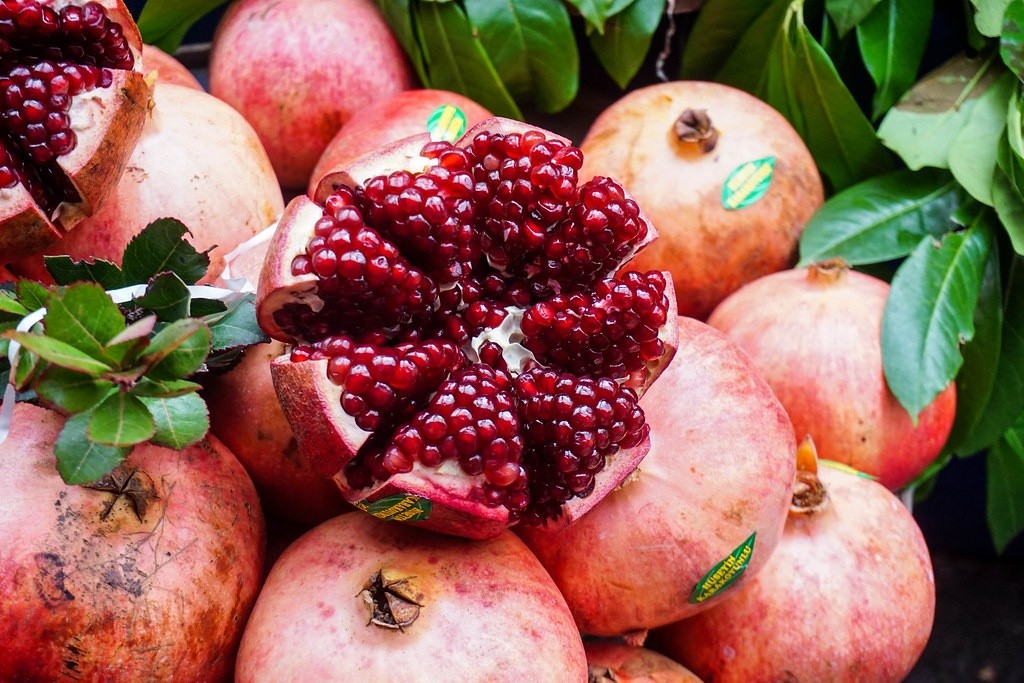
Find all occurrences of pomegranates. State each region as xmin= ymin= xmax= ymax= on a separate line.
xmin=710 ymin=264 xmax=954 ymax=485
xmin=581 ymin=639 xmax=706 ymax=683
xmin=140 ymin=43 xmax=205 ymax=91
xmin=5 ymin=82 xmax=286 ymax=295
xmin=254 ymin=129 xmax=678 ymax=541
xmin=524 ymin=310 xmax=796 ymax=636
xmin=0 ymin=403 xmax=262 ymax=683
xmin=208 ymin=0 xmax=411 ymax=188
xmin=217 ymin=504 xmax=589 ymax=641
xmin=306 ymin=90 xmax=491 ymax=198
xmin=580 ymin=83 xmax=825 ymax=317
xmin=0 ymin=0 xmax=155 ymax=255
xmin=656 ymin=436 xmax=936 ymax=683
